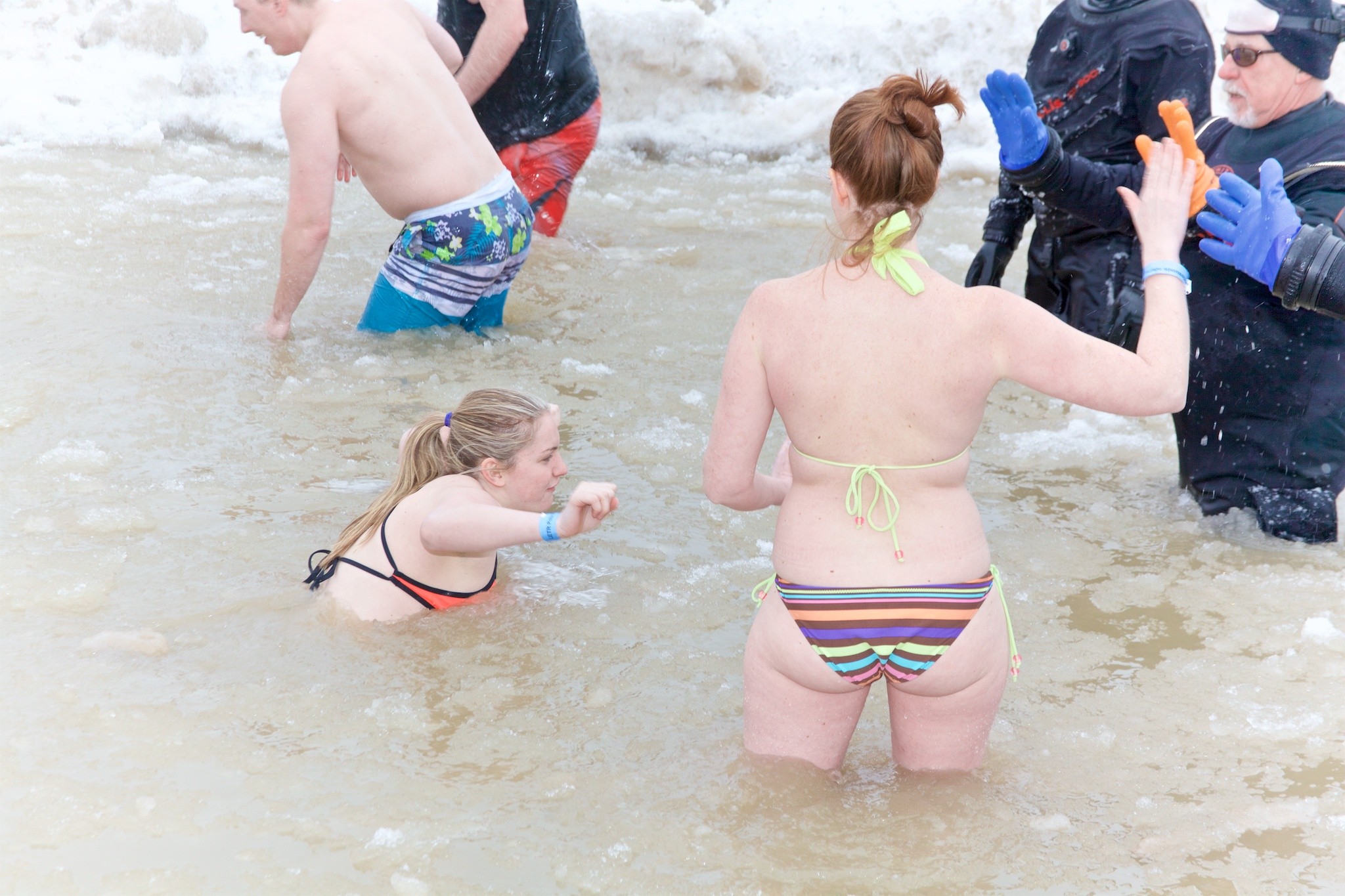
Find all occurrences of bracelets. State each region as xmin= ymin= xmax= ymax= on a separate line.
xmin=539 ymin=512 xmax=563 ymax=543
xmin=1142 ymin=259 xmax=1192 ymax=296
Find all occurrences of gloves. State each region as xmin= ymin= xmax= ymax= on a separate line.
xmin=1135 ymin=100 xmax=1220 ymax=218
xmin=1196 ymin=157 xmax=1303 ymax=295
xmin=980 ymin=68 xmax=1049 ymax=171
xmin=964 ymin=240 xmax=1012 ymax=288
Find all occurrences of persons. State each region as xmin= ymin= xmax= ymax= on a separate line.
xmin=429 ymin=0 xmax=605 ymax=240
xmin=979 ymin=1 xmax=1344 ymax=544
xmin=963 ymin=0 xmax=1217 ymax=358
xmin=702 ymin=69 xmax=1202 ymax=775
xmin=233 ymin=1 xmax=537 ymax=348
xmin=300 ymin=385 xmax=623 ymax=624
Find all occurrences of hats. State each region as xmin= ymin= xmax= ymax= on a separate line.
xmin=1255 ymin=0 xmax=1345 ymax=80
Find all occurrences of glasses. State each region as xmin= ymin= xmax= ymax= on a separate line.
xmin=1219 ymin=41 xmax=1279 ymax=66
